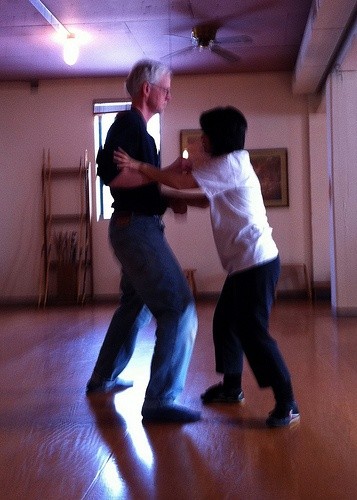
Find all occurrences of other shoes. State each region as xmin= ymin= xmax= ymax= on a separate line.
xmin=142 ymin=404 xmax=202 ymax=424
xmin=86 ymin=376 xmax=133 ymax=392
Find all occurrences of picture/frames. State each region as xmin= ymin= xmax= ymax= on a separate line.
xmin=245 ymin=148 xmax=289 ymax=208
xmin=179 ymin=128 xmax=204 ymax=163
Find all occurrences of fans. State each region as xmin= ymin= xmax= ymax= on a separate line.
xmin=159 ymin=22 xmax=254 ymax=65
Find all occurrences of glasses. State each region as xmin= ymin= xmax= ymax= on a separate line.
xmin=150 ymin=83 xmax=171 ymax=96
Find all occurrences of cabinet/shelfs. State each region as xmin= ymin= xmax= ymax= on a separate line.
xmin=38 ymin=148 xmax=95 ymax=306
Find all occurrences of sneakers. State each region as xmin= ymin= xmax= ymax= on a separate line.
xmin=266 ymin=401 xmax=300 ymax=426
xmin=201 ymin=382 xmax=245 ymax=401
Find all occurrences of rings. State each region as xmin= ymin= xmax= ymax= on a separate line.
xmin=189 ymin=164 xmax=192 ymax=168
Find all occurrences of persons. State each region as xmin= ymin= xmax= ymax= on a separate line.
xmin=112 ymin=105 xmax=302 ymax=430
xmin=85 ymin=60 xmax=203 ymax=425
